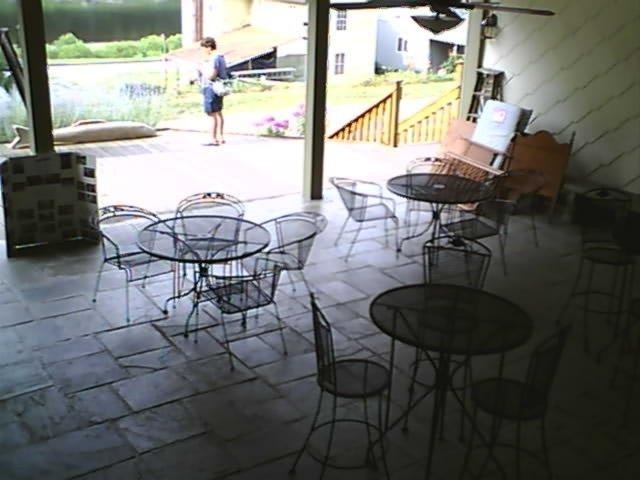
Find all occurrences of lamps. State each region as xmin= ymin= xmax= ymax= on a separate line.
xmin=480 ymin=13 xmax=500 ymax=39
xmin=411 ymin=14 xmax=465 ymax=35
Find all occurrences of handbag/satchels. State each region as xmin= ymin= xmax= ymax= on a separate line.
xmin=210 ymin=77 xmax=232 ymax=97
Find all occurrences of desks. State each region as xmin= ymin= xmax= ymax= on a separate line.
xmin=137 ymin=215 xmax=271 ymax=339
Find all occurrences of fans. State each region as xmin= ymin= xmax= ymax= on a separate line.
xmin=329 ymin=0 xmax=555 ymax=16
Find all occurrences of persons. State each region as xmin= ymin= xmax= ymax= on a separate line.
xmin=199 ymin=37 xmax=232 ymax=145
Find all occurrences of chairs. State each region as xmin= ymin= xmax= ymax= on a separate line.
xmin=172 ymin=192 xmax=246 ymax=298
xmin=241 ymin=211 xmax=328 ymax=319
xmin=193 ymin=257 xmax=289 ymax=372
xmin=89 ymin=205 xmax=177 ymax=322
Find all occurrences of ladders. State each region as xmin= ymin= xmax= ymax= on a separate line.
xmin=465 ymin=67 xmax=505 ymax=123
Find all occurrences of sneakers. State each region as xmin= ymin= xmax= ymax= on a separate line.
xmin=203 ymin=140 xmax=220 ymax=146
